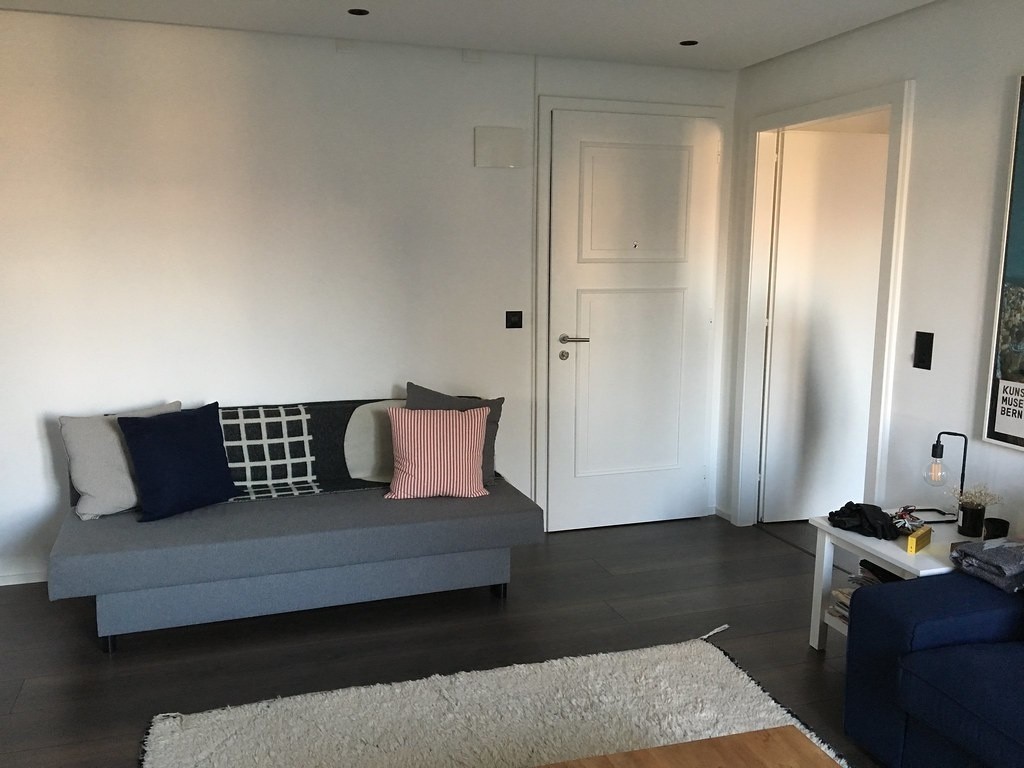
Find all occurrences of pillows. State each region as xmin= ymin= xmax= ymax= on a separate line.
xmin=403 ymin=383 xmax=504 ymax=488
xmin=115 ymin=401 xmax=247 ymax=525
xmin=382 ymin=405 xmax=490 ymax=500
xmin=342 ymin=400 xmax=412 ymax=484
xmin=56 ymin=401 xmax=183 ymax=521
xmin=216 ymin=406 xmax=323 ymax=497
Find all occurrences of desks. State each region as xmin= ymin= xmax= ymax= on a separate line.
xmin=809 ymin=511 xmax=1012 ymax=649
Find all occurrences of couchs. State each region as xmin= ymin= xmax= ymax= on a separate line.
xmin=842 ymin=570 xmax=1024 ymax=768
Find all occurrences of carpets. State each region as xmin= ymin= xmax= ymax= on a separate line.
xmin=141 ymin=640 xmax=853 ymax=768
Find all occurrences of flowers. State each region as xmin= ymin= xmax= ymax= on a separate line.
xmin=949 ymin=485 xmax=1004 ymax=510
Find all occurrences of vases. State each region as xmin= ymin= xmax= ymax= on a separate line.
xmin=957 ymin=504 xmax=986 ymax=538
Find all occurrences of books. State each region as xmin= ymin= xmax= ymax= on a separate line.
xmin=827 ymin=588 xmax=857 ymax=625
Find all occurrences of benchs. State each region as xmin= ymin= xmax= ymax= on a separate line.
xmin=45 ymin=397 xmax=544 ymax=652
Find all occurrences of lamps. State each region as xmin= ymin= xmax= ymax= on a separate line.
xmin=905 ymin=432 xmax=967 ymax=524
